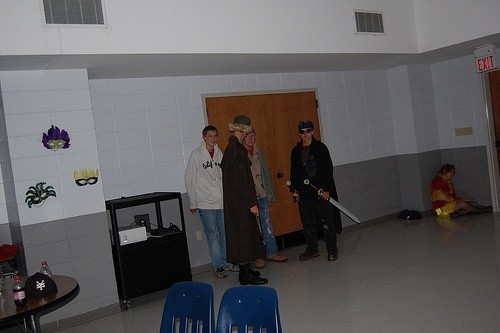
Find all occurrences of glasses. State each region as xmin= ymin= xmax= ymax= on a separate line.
xmin=300 ymin=128 xmax=312 ymax=134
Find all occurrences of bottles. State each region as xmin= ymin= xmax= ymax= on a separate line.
xmin=0 ymin=265 xmax=5 ymax=291
xmin=13 ymin=276 xmax=26 ymax=306
xmin=41 ymin=261 xmax=53 ymax=279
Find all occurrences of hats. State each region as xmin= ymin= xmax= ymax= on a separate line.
xmin=25 ymin=272 xmax=58 ymax=296
xmin=234 ymin=115 xmax=251 ymax=126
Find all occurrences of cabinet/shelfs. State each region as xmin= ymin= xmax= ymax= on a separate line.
xmin=105 ymin=192 xmax=193 ymax=309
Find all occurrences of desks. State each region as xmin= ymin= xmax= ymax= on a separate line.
xmin=0 ymin=274 xmax=79 ymax=333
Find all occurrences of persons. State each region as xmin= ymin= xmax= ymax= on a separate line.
xmin=241 ymin=128 xmax=289 ymax=270
xmin=430 ymin=163 xmax=492 ymax=217
xmin=221 ymin=115 xmax=268 ymax=285
xmin=184 ymin=125 xmax=240 ymax=278
xmin=290 ymin=121 xmax=342 ymax=261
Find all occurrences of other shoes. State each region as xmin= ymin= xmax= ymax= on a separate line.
xmin=248 ymin=269 xmax=261 ymax=276
xmin=449 ymin=212 xmax=459 ymax=219
xmin=255 ymin=258 xmax=265 ymax=269
xmin=223 ymin=263 xmax=240 ymax=272
xmin=328 ymin=254 xmax=337 ymax=261
xmin=299 ymin=252 xmax=320 ymax=261
xmin=214 ymin=267 xmax=227 ymax=279
xmin=267 ymin=254 xmax=288 ymax=262
xmin=239 ymin=270 xmax=268 ymax=286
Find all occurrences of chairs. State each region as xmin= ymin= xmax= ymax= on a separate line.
xmin=160 ymin=281 xmax=282 ymax=333
